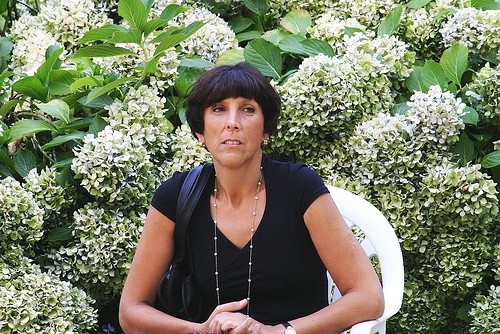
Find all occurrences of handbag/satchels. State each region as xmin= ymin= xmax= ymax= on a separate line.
xmin=158 ymin=162 xmax=213 ymax=325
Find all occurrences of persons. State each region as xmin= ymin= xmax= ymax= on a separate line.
xmin=116 ymin=61 xmax=386 ymax=334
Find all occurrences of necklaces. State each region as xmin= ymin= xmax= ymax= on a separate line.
xmin=209 ymin=154 xmax=264 ymax=318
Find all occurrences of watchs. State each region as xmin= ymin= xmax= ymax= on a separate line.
xmin=280 ymin=321 xmax=297 ymax=334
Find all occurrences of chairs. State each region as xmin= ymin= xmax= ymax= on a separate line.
xmin=323 ymin=184 xmax=404 ymax=334
